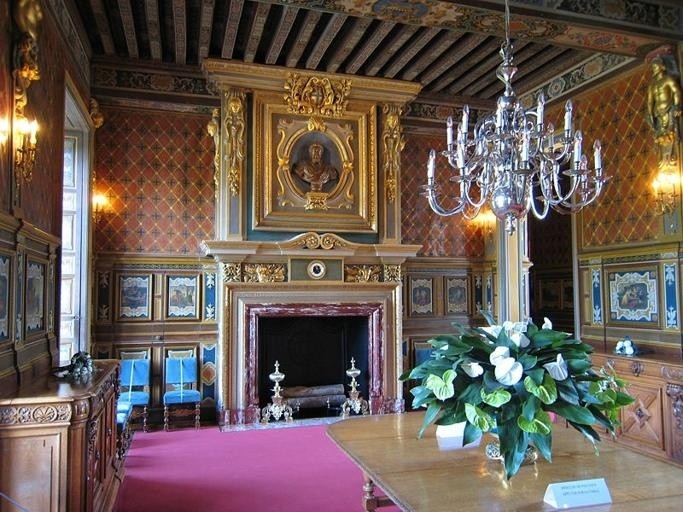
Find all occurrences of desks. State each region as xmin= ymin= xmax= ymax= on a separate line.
xmin=326 ymin=410 xmax=683 ymax=512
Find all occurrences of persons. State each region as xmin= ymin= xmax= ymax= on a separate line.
xmin=647 ymin=57 xmax=682 ymax=136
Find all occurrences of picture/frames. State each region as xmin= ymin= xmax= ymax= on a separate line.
xmin=601 ymin=261 xmax=663 ymax=330
xmin=1 ymin=246 xmax=49 ymax=348
xmin=408 ymin=274 xmax=472 ymax=319
xmin=112 ymin=269 xmax=202 ymax=322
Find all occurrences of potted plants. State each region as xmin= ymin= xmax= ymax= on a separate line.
xmin=396 ymin=309 xmax=639 ymax=483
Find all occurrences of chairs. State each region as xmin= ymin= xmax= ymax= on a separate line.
xmin=116 ymin=357 xmax=201 ymax=461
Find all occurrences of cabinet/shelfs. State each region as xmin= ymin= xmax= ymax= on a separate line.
xmin=0 ymin=359 xmax=128 ymax=512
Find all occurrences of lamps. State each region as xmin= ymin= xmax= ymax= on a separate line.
xmin=416 ymin=2 xmax=616 ymax=235
